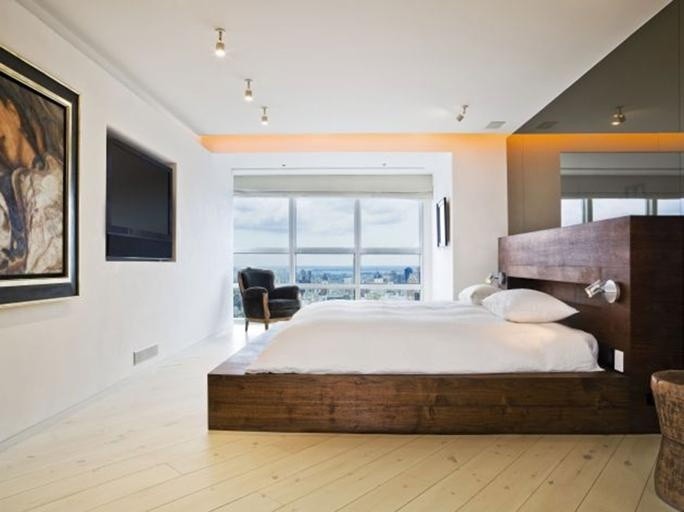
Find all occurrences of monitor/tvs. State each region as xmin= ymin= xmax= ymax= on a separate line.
xmin=105 ymin=135 xmax=174 ymax=262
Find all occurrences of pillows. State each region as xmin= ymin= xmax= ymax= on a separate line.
xmin=459 ymin=284 xmax=580 ymax=324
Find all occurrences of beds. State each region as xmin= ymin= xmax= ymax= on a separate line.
xmin=276 ymin=297 xmax=605 ymax=372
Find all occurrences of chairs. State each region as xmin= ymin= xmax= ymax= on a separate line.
xmin=236 ymin=267 xmax=301 ymax=333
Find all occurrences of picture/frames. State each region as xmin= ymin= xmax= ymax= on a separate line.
xmin=1 ymin=46 xmax=81 ymax=309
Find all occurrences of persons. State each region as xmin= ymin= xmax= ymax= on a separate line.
xmin=0 ymin=77 xmax=66 ymax=274
